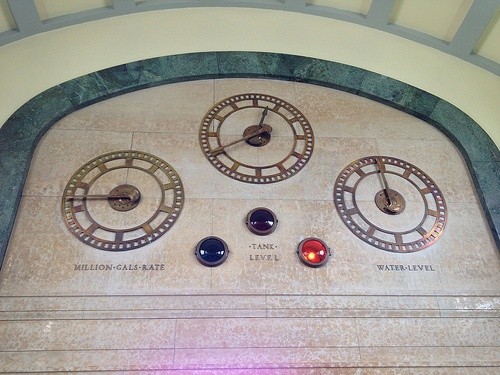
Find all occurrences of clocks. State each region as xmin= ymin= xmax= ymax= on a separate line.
xmin=198 ymin=92 xmax=315 ymax=185
xmin=333 ymin=155 xmax=447 ymax=253
xmin=61 ymin=149 xmax=184 ymax=252
